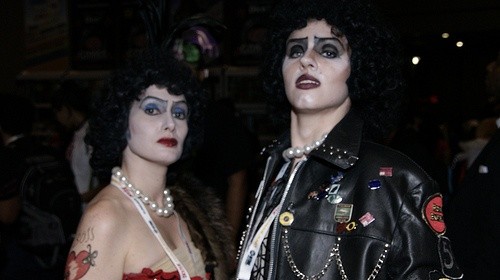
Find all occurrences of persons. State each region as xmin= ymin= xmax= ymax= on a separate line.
xmin=192 ymin=112 xmax=249 ymax=246
xmin=64 ymin=47 xmax=234 ymax=280
xmin=36 ymin=72 xmax=87 ymax=155
xmin=230 ymin=1 xmax=467 ymax=280
xmin=0 ymin=76 xmax=82 ymax=280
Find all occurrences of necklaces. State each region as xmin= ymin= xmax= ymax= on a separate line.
xmin=112 ymin=167 xmax=175 ymax=217
xmin=282 ymin=133 xmax=328 ymax=159
